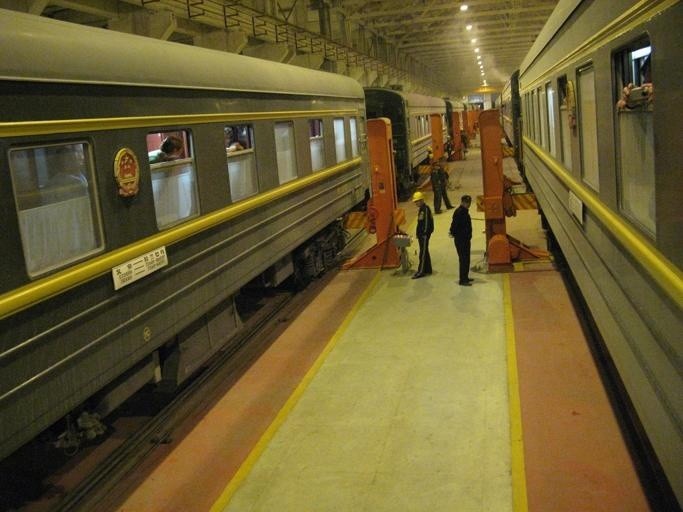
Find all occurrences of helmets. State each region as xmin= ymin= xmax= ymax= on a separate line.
xmin=412 ymin=191 xmax=424 ymax=203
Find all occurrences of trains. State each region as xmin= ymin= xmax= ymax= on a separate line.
xmin=0 ymin=10 xmax=477 ymax=467
xmin=496 ymin=0 xmax=683 ymax=512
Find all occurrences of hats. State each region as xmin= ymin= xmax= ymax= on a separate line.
xmin=461 ymin=195 xmax=472 ymax=202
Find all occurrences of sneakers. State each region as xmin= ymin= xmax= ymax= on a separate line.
xmin=459 ymin=279 xmax=474 ymax=286
xmin=434 ymin=206 xmax=454 ymax=214
xmin=412 ymin=269 xmax=432 ymax=279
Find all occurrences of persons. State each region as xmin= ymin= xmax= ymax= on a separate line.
xmin=410 ymin=191 xmax=434 ymax=281
xmin=431 ymin=166 xmax=445 ymax=216
xmin=48 ymin=149 xmax=88 ymax=190
xmin=432 ymin=162 xmax=455 ymax=211
xmin=149 ymin=137 xmax=184 ymax=163
xmin=447 ymin=194 xmax=475 ymax=287
xmin=447 ymin=141 xmax=452 ymax=158
xmin=462 ymin=134 xmax=467 ymax=150
xmin=616 ymin=53 xmax=653 ymax=111
xmin=224 ymin=130 xmax=245 ymax=154
